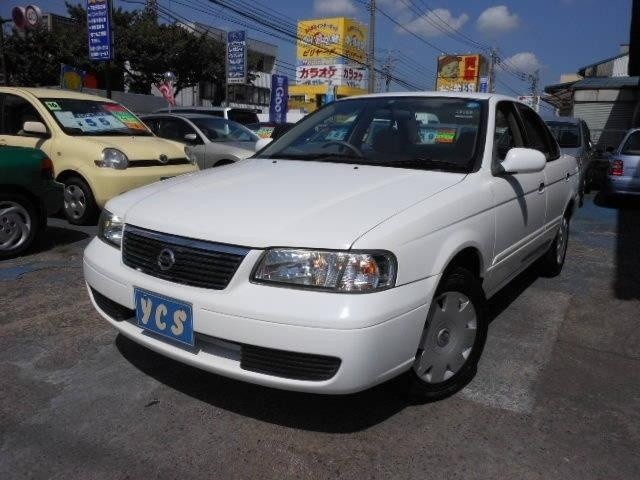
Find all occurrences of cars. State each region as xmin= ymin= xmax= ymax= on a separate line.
xmin=605 ymin=128 xmax=639 ymax=197
xmin=83 ymin=90 xmax=583 ymax=405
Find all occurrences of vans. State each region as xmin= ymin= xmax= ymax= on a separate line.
xmin=544 ymin=109 xmax=595 ymax=185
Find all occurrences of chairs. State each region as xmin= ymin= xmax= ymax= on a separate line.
xmin=361 ymin=125 xmax=483 ymax=163
